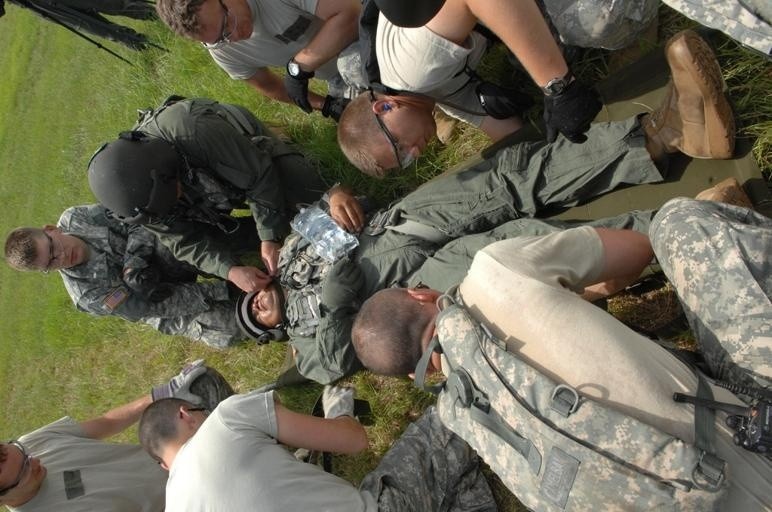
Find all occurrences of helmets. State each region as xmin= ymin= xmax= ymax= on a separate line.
xmin=88 ymin=132 xmax=177 ymax=225
xmin=234 ymin=290 xmax=286 ymax=345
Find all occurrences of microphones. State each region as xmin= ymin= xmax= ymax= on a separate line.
xmin=182 ymin=155 xmax=199 ymax=185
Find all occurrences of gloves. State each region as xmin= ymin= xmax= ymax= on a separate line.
xmin=151 ymin=359 xmax=207 ymax=404
xmin=322 ymin=95 xmax=351 ymax=123
xmin=322 ymin=384 xmax=355 ymax=420
xmin=284 ymin=57 xmax=314 ymax=115
xmin=542 ymin=73 xmax=602 ymax=144
xmin=322 ymin=258 xmax=364 ymax=310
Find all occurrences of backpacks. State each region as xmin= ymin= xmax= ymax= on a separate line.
xmin=413 ymin=285 xmax=727 ymax=511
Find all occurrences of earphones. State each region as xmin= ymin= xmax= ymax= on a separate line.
xmin=384 ymin=104 xmax=391 ymax=111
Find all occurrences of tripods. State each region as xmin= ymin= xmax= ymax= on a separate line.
xmin=24 ymin=0 xmax=173 ymax=72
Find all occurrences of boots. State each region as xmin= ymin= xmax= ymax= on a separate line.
xmin=640 ymin=30 xmax=735 ymax=160
xmin=694 ymin=177 xmax=753 ymax=210
xmin=432 ymin=110 xmax=468 ymax=145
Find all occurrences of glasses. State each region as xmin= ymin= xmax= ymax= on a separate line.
xmin=366 ymin=86 xmax=409 ymax=177
xmin=0 ymin=440 xmax=29 ymax=500
xmin=42 ymin=232 xmax=59 ymax=274
xmin=200 ymin=0 xmax=234 ymax=49
xmin=181 ymin=407 xmax=211 ymax=418
